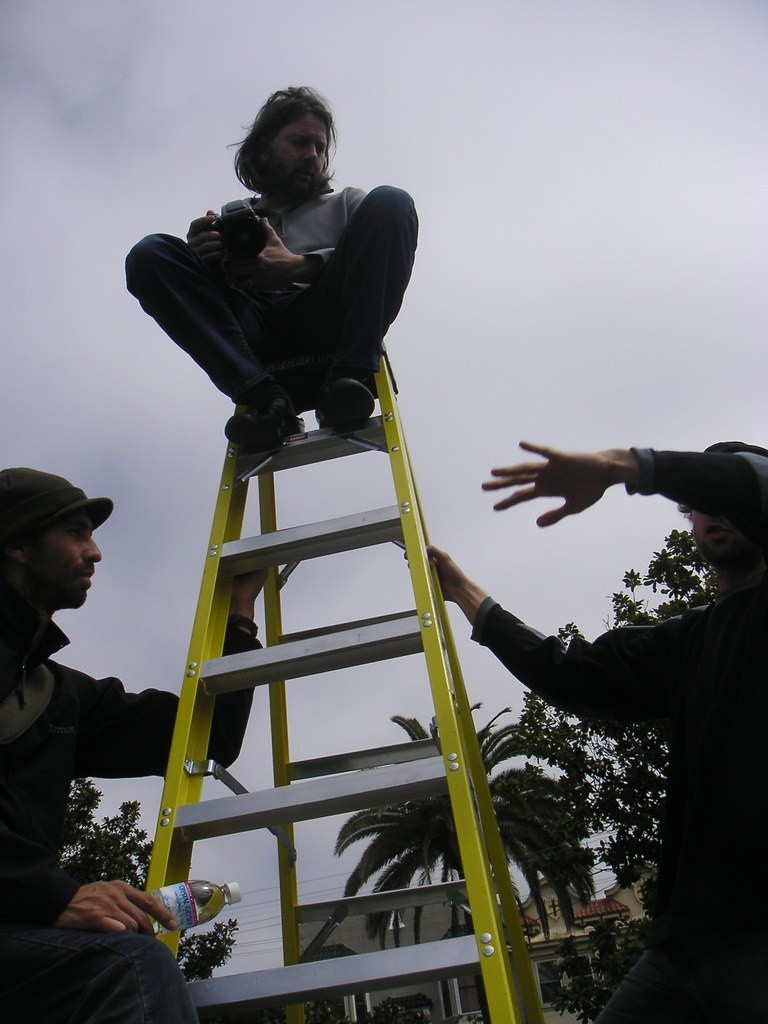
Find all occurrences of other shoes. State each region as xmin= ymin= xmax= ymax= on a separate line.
xmin=225 ymin=392 xmax=305 ymax=445
xmin=315 ymin=374 xmax=375 ymax=429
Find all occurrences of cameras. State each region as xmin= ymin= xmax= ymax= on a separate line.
xmin=204 ymin=203 xmax=268 ymax=259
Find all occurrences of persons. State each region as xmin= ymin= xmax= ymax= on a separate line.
xmin=126 ymin=86 xmax=419 ymax=446
xmin=0 ymin=467 xmax=269 ymax=1024
xmin=403 ymin=442 xmax=768 ymax=1023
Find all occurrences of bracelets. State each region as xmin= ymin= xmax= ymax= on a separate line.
xmin=228 ymin=614 xmax=257 ymax=636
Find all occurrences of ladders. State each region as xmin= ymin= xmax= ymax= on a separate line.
xmin=139 ymin=339 xmax=549 ymax=1022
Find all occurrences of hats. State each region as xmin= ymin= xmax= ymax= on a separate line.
xmin=0 ymin=468 xmax=114 ymax=549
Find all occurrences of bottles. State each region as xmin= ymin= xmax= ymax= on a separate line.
xmin=145 ymin=880 xmax=241 ymax=934
